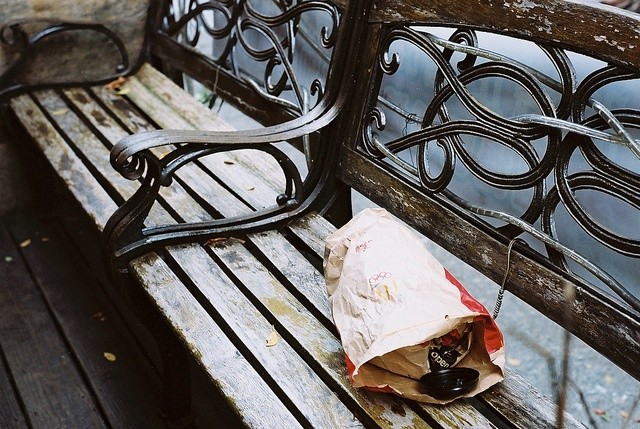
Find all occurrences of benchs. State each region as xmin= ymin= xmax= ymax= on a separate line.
xmin=107 ymin=0 xmax=640 ymax=429
xmin=0 ymin=0 xmax=362 ymax=247
